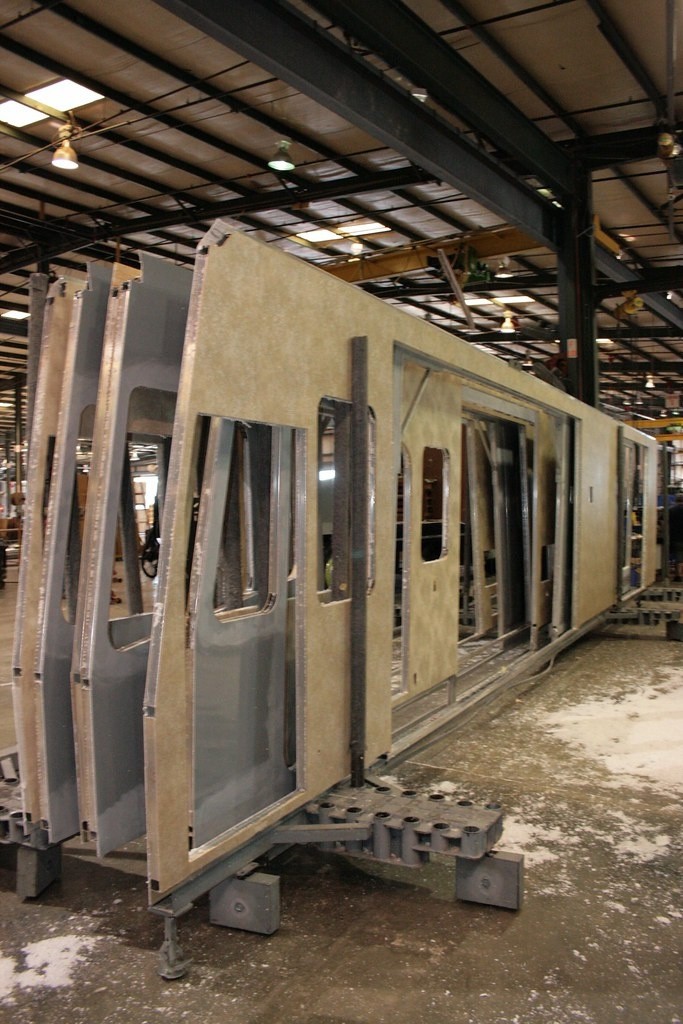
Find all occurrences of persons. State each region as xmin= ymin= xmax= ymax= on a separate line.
xmin=668 ymin=493 xmax=683 ymax=582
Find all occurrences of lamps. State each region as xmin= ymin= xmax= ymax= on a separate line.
xmin=614 ymin=290 xmax=644 ymax=319
xmin=501 ymin=310 xmax=517 ymax=333
xmin=51 ymin=124 xmax=81 ymax=170
xmin=493 ymin=262 xmax=514 ymax=279
xmin=658 ymin=134 xmax=682 ymax=161
xmin=268 ymin=140 xmax=296 ymax=171
